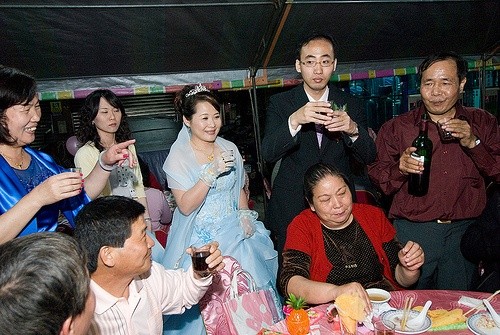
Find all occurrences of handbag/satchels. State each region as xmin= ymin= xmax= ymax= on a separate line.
xmin=221 ymin=267 xmax=279 ymax=335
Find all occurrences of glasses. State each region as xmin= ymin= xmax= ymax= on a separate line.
xmin=301 ymin=58 xmax=336 ymax=67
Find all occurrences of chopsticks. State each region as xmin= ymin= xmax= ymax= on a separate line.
xmin=463 ymin=290 xmax=500 ymax=318
xmin=401 ymin=297 xmax=414 ymax=329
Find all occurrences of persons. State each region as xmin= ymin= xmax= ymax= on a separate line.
xmin=366 ymin=51 xmax=500 ymax=291
xmin=159 ymin=83 xmax=279 ymax=335
xmin=75 ymin=196 xmax=225 ymax=335
xmin=145 ymin=187 xmax=172 ymax=231
xmin=0 ymin=231 xmax=96 ymax=335
xmin=0 ymin=65 xmax=136 ymax=245
xmin=261 ymin=32 xmax=377 ymax=298
xmin=279 ymin=163 xmax=424 ymax=317
xmin=74 ymin=89 xmax=165 ymax=264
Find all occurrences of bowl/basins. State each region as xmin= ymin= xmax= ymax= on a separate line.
xmin=365 ymin=288 xmax=391 ymax=303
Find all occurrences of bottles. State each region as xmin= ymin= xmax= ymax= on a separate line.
xmin=408 ymin=114 xmax=433 ymax=197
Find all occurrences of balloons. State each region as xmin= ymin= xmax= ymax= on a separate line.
xmin=66 ymin=136 xmax=84 ymax=156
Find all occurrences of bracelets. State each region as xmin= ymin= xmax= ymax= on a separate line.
xmin=475 ymin=138 xmax=481 ymax=146
xmin=99 ymin=150 xmax=116 ymax=170
xmin=145 ymin=218 xmax=151 ymax=222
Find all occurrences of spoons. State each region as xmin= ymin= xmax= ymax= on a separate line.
xmin=406 ymin=300 xmax=432 ymax=329
xmin=483 ymin=299 xmax=500 ymax=327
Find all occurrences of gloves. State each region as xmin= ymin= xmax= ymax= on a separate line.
xmin=237 ymin=210 xmax=260 ymax=241
xmin=199 ymin=151 xmax=236 ymax=190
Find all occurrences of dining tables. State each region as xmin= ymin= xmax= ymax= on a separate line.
xmin=264 ymin=290 xmax=500 ymax=335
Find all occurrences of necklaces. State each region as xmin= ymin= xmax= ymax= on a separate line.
xmin=191 ymin=141 xmax=215 ymax=161
xmin=0 ymin=148 xmax=25 ymax=169
xmin=98 ymin=139 xmax=115 ymax=148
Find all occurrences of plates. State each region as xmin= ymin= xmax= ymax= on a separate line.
xmin=466 ymin=312 xmax=500 ymax=335
xmin=380 ymin=309 xmax=432 ymax=334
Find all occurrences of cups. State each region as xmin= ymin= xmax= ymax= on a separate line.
xmin=62 ymin=168 xmax=82 ymax=191
xmin=374 ymin=320 xmax=395 ymax=335
xmin=190 ymin=242 xmax=211 ymax=273
xmin=225 ymin=150 xmax=234 ymax=168
xmin=438 ymin=117 xmax=454 ymax=141
xmin=315 ymin=101 xmax=331 ymax=126
